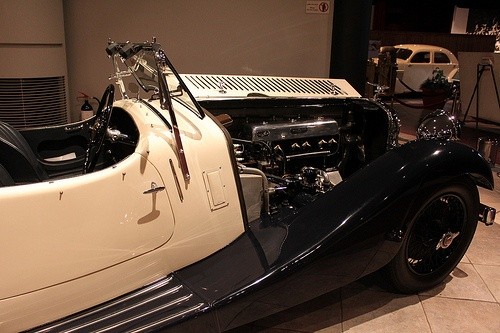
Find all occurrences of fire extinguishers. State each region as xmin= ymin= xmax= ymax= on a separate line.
xmin=77 ymin=91 xmax=93 ymax=121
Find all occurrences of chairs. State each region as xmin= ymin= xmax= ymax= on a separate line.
xmin=0 ymin=122 xmax=87 ymax=183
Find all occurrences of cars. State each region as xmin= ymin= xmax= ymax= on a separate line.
xmin=369 ymin=44 xmax=460 ymax=96
xmin=1 ymin=38 xmax=500 ymax=333
